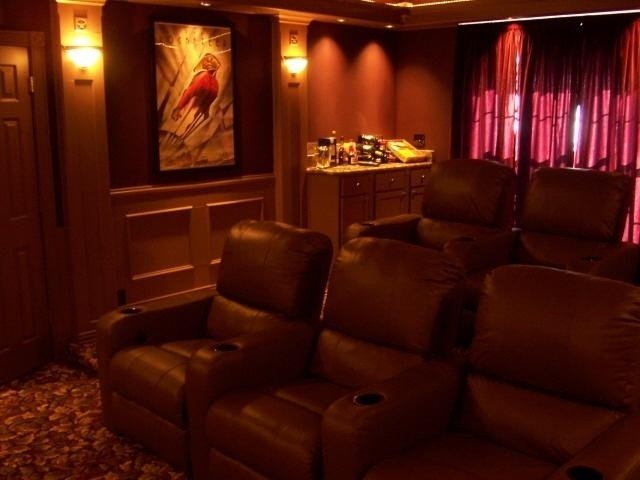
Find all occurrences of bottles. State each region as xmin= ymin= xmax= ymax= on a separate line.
xmin=316 ymin=130 xmax=389 ymax=169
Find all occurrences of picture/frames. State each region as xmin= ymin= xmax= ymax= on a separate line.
xmin=148 ymin=12 xmax=241 ymax=180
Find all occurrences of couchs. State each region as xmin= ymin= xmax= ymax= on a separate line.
xmin=95 ymin=219 xmax=333 ymax=474
xmin=343 ymin=159 xmax=521 ymax=268
xmin=182 ymin=236 xmax=481 ymax=480
xmin=321 ymin=263 xmax=639 ymax=480
xmin=451 ymin=167 xmax=640 ymax=285
